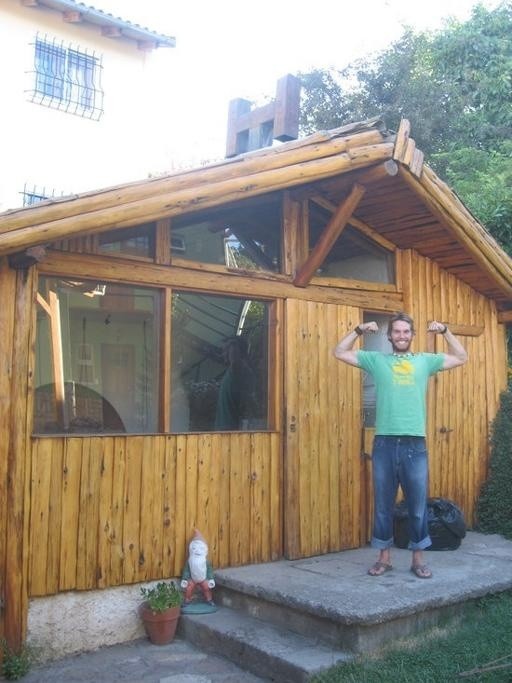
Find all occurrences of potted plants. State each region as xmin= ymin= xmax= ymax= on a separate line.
xmin=140 ymin=581 xmax=182 ymax=646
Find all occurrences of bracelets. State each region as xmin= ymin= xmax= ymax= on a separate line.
xmin=353 ymin=325 xmax=364 ymax=335
xmin=440 ymin=324 xmax=447 ymax=333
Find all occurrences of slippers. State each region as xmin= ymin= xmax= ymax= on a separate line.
xmin=410 ymin=562 xmax=434 ymax=578
xmin=367 ymin=562 xmax=393 ymax=576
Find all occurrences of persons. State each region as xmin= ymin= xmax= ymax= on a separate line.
xmin=211 ymin=333 xmax=259 ymax=430
xmin=331 ymin=316 xmax=471 ymax=579
xmin=179 ymin=529 xmax=216 ymax=609
